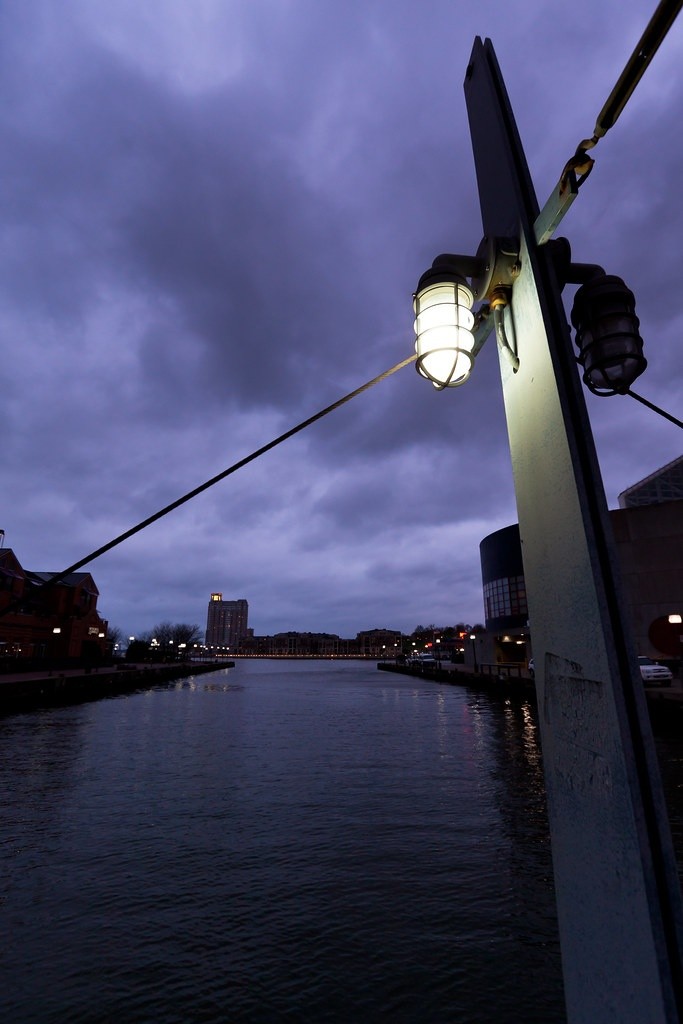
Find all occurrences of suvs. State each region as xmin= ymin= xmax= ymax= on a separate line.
xmin=637 ymin=654 xmax=673 ymax=687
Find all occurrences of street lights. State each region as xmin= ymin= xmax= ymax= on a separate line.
xmin=469 ymin=633 xmax=478 ymax=673
xmin=381 ymin=639 xmax=428 ymax=669
xmin=45 ymin=625 xmax=229 ymax=677
xmin=408 ymin=31 xmax=682 ymax=1024
xmin=435 ymin=636 xmax=442 ymax=671
